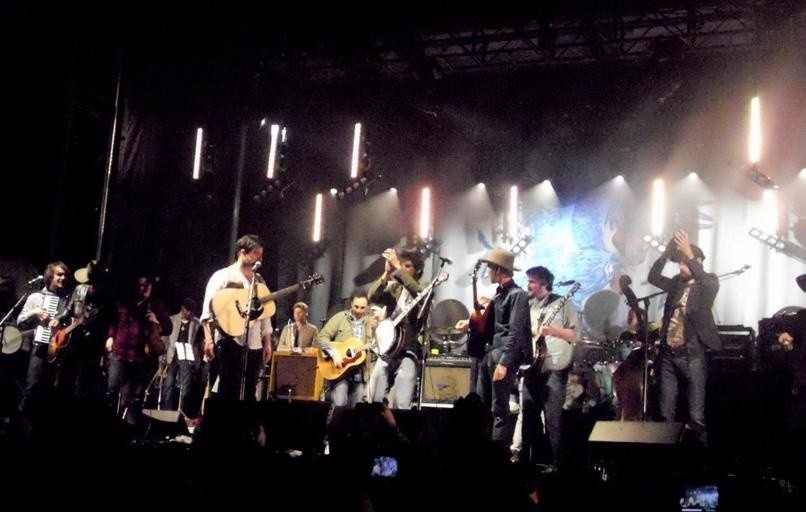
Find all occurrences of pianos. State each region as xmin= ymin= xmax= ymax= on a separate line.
xmin=425 ymin=355 xmax=473 ymax=367
xmin=715 ymin=326 xmax=756 ymax=360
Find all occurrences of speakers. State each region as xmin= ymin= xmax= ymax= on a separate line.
xmin=757 ymin=314 xmax=806 ymax=372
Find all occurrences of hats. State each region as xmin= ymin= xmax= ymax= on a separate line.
xmin=670 ymin=243 xmax=707 ymax=263
xmin=183 ymin=297 xmax=198 ymax=313
xmin=481 ymin=248 xmax=521 ymax=273
xmin=75 ymin=261 xmax=110 ymax=284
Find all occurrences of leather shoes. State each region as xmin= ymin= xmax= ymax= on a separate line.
xmin=540 ymin=465 xmax=560 ymax=477
xmin=511 ymin=450 xmax=522 ymax=466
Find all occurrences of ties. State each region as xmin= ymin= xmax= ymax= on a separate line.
xmin=295 ymin=328 xmax=299 ymax=346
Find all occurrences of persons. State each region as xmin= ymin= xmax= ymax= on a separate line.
xmin=524 ymin=267 xmax=581 ymax=476
xmin=199 ymin=234 xmax=273 ymax=446
xmin=619 ymin=308 xmax=647 ymax=360
xmin=368 ymin=248 xmax=435 ymax=445
xmin=105 ymin=276 xmax=173 ymax=441
xmin=276 ymin=302 xmax=320 ymax=350
xmin=4 ymin=263 xmax=70 ymax=428
xmin=469 ymin=297 xmax=493 ymax=393
xmin=648 ymin=228 xmax=719 ymax=447
xmin=50 ymin=260 xmax=101 ymax=430
xmin=456 ymin=249 xmax=534 ymax=450
xmin=317 ymin=290 xmax=374 ymax=454
xmin=161 ymin=298 xmax=205 ymax=421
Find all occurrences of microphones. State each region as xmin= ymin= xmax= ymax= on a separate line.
xmin=556 ymin=279 xmax=577 ymax=289
xmin=251 ymin=260 xmax=262 ymax=273
xmin=439 ymin=256 xmax=454 ymax=265
xmin=288 ymin=318 xmax=292 ymax=325
xmin=26 ymin=275 xmax=43 ymax=285
xmin=736 ymin=264 xmax=751 ymax=276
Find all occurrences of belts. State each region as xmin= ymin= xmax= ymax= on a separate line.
xmin=662 ymin=348 xmax=689 ymax=358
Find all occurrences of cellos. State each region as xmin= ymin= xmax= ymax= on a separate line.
xmin=611 ymin=276 xmax=662 ymax=421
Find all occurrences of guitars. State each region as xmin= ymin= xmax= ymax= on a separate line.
xmin=318 ymin=338 xmax=371 ymax=382
xmin=210 ymin=272 xmax=326 ymax=339
xmin=47 ymin=306 xmax=99 ymax=364
xmin=466 ymin=262 xmax=495 ymax=358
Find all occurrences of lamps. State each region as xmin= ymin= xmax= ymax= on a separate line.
xmin=748 ymin=227 xmax=785 ymax=251
xmin=350 ymin=123 xmax=362 ymax=179
xmin=727 ymin=157 xmax=777 ymax=189
xmin=266 ymin=121 xmax=279 ymax=179
xmin=642 ymin=233 xmax=666 ymax=253
xmin=335 ymin=171 xmax=374 ymax=201
xmin=313 ymin=193 xmax=322 ymax=242
xmin=193 ymin=127 xmax=204 ymax=179
xmin=252 ymin=179 xmax=283 ymax=206
xmin=510 ymin=186 xmax=518 ymax=236
xmin=747 ymin=97 xmax=763 ymax=164
xmin=510 ymin=236 xmax=531 ymax=256
xmin=420 ymin=187 xmax=430 ymax=240
